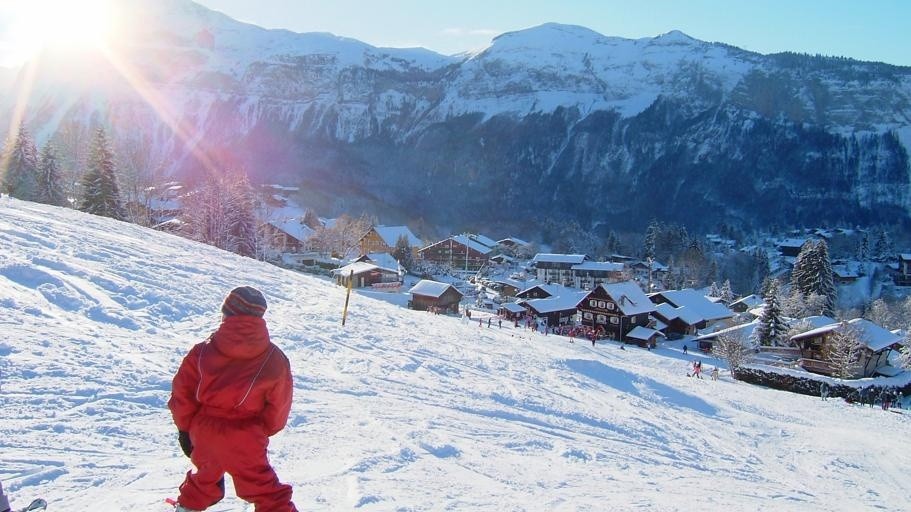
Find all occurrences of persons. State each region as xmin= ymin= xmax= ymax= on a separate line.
xmin=683 ymin=345 xmax=687 ymax=355
xmin=167 ymin=286 xmax=298 ymax=512
xmin=479 ymin=317 xmax=502 ymax=329
xmin=544 ymin=322 xmax=602 ymax=346
xmin=692 ymin=361 xmax=719 ymax=381
xmin=861 ymin=388 xmax=903 ymax=410
xmin=820 ymin=382 xmax=828 ymax=401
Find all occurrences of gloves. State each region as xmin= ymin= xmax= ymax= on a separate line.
xmin=177 ymin=431 xmax=190 ymax=457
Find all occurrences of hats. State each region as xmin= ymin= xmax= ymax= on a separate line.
xmin=221 ymin=285 xmax=267 ymax=318
